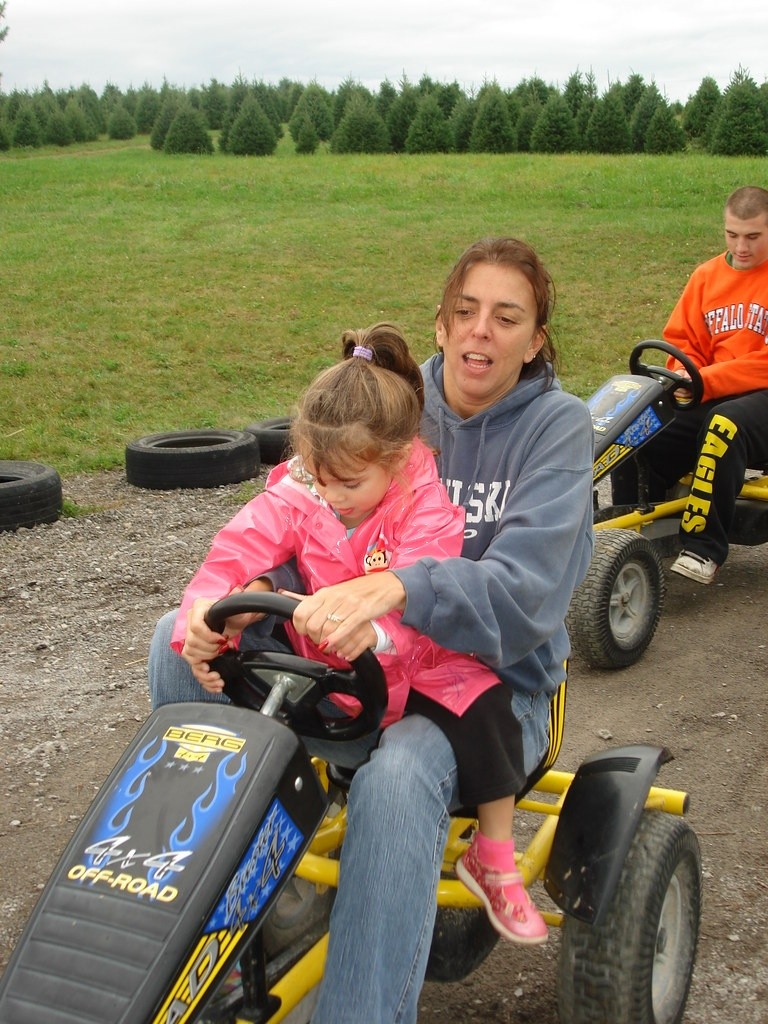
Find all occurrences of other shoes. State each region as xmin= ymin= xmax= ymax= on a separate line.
xmin=454 ymin=843 xmax=549 ymax=946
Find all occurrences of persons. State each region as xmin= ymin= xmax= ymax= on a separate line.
xmin=147 ymin=237 xmax=595 ymax=1024
xmin=610 ymin=185 xmax=768 ymax=585
xmin=168 ymin=322 xmax=548 ymax=945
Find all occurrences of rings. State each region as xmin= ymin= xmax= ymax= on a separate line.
xmin=328 ymin=613 xmax=343 ymax=622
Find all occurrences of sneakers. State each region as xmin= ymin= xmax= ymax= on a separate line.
xmin=669 ymin=549 xmax=718 ymax=584
